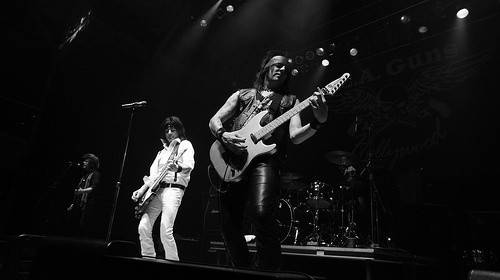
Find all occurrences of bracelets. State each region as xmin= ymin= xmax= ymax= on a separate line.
xmin=216 ymin=128 xmax=226 ymax=141
xmin=310 ymin=119 xmax=322 ymax=130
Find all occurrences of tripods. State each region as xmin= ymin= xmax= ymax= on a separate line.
xmin=298 ymin=156 xmax=371 ymax=247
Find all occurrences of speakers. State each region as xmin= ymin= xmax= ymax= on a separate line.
xmin=0 ymin=234 xmax=325 ymax=280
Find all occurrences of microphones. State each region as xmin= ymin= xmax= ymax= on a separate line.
xmin=69 ymin=162 xmax=80 ymax=166
xmin=122 ymin=101 xmax=147 ymax=109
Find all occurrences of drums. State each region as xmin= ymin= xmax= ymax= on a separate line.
xmin=312 ymin=211 xmax=332 ymax=247
xmin=279 ymin=198 xmax=310 ymax=245
xmin=336 ymin=176 xmax=369 ymax=212
xmin=305 ymin=180 xmax=333 ymax=209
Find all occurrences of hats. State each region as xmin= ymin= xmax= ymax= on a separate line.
xmin=80 ymin=153 xmax=99 ymax=169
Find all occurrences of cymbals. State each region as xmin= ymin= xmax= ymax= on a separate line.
xmin=280 ymin=179 xmax=307 ymax=191
xmin=277 ymin=173 xmax=305 ymax=179
xmin=325 ymin=150 xmax=353 ymax=166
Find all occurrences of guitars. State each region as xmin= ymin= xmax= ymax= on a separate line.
xmin=209 ymin=72 xmax=352 ymax=184
xmin=134 ymin=148 xmax=188 ymax=220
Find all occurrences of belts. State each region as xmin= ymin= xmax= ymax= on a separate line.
xmin=161 ymin=183 xmax=185 ymax=190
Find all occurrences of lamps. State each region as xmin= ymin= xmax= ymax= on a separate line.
xmin=286 ymin=40 xmax=358 ymax=78
xmin=215 ymin=1 xmax=236 ymax=18
xmin=456 ymin=0 xmax=469 ymax=20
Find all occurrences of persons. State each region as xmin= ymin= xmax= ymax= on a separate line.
xmin=67 ymin=154 xmax=100 ymax=230
xmin=131 ymin=116 xmax=195 ymax=262
xmin=209 ymin=52 xmax=330 ymax=270
